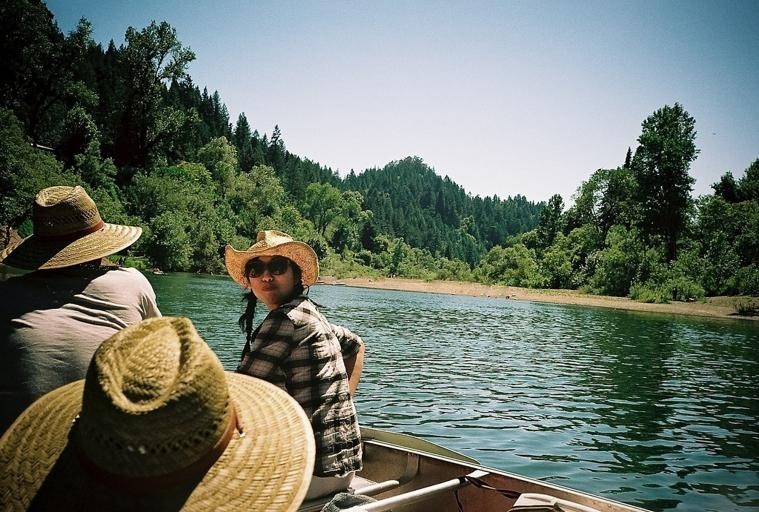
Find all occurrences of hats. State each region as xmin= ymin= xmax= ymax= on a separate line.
xmin=0 ymin=184 xmax=143 ymax=271
xmin=225 ymin=229 xmax=321 ymax=290
xmin=1 ymin=315 xmax=318 ymax=512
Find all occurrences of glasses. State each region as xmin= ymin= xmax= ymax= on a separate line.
xmin=244 ymin=258 xmax=288 ymax=278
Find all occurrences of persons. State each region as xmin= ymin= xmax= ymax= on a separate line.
xmin=0 ymin=185 xmax=162 ymax=439
xmin=225 ymin=231 xmax=364 ymax=500
